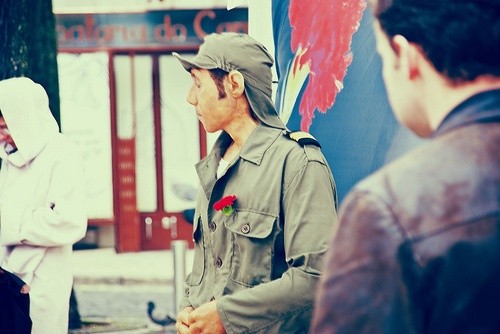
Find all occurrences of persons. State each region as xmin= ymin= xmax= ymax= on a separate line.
xmin=172 ymin=31 xmax=339 ymax=334
xmin=0 ymin=77 xmax=88 ymax=334
xmin=310 ymin=0 xmax=500 ymax=334
